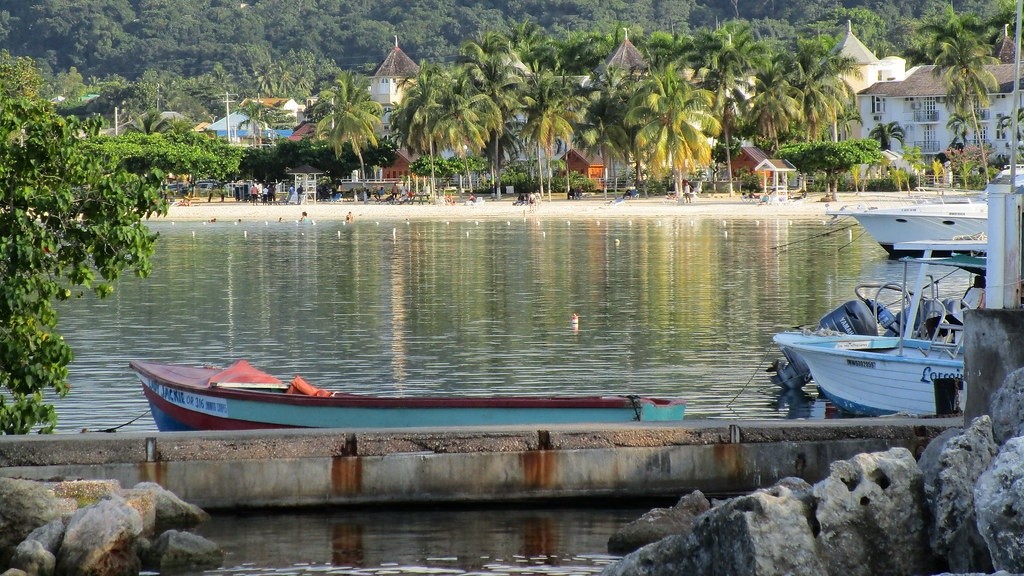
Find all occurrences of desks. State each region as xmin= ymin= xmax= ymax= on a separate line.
xmin=409 ymin=194 xmax=430 ymax=205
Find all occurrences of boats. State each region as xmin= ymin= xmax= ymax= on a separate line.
xmin=127 ymin=355 xmax=688 ymax=437
xmin=825 ymin=201 xmax=989 ymax=259
xmin=766 ymin=241 xmax=987 ymax=418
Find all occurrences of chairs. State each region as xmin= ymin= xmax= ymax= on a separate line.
xmin=608 ymin=199 xmax=626 ymax=210
xmin=267 ymin=195 xmax=287 ymax=206
xmin=323 ymin=192 xmax=342 ymax=203
xmin=625 ymin=193 xmax=640 ymax=201
xmin=472 ymin=197 xmax=485 ymax=210
xmin=275 ymin=195 xmax=293 ymax=205
xmin=664 ymin=188 xmax=803 ymax=209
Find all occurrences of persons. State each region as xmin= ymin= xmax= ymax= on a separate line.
xmin=299 ymin=211 xmax=310 ymax=223
xmin=221 ymin=193 xmax=224 ymax=202
xmin=250 ymin=182 xmax=760 ymax=206
xmin=208 ymin=191 xmax=213 ymax=201
xmin=279 ymin=218 xmax=285 ymax=222
xmin=158 ymin=176 xmax=166 ymax=205
xmin=211 ymin=218 xmax=242 ymax=223
xmin=346 ymin=212 xmax=353 ymax=222
